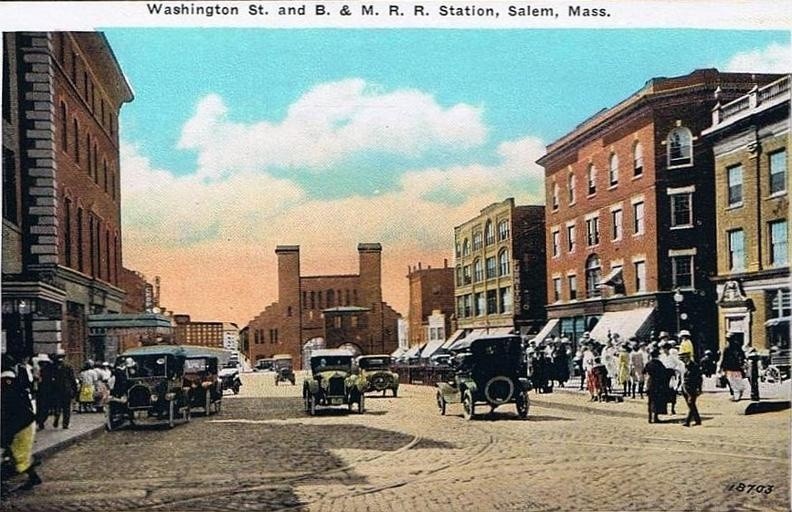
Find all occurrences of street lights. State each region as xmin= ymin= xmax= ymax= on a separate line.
xmin=483 ymin=318 xmax=491 ymax=336
xmin=674 ymin=287 xmax=687 ymax=345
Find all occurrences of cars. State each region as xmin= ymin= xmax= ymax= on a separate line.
xmin=254 ymin=358 xmax=274 ymax=372
xmin=301 ymin=351 xmax=363 ymax=416
xmin=434 ymin=333 xmax=533 ymax=421
xmin=182 ymin=355 xmax=224 ymax=417
xmin=103 ymin=345 xmax=194 ymax=432
xmin=217 ymin=365 xmax=242 ymax=396
xmin=353 ymin=353 xmax=400 ymax=397
xmin=272 ymin=353 xmax=296 ymax=386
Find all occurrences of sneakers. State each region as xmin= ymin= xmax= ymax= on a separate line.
xmin=692 ymin=422 xmax=701 ymax=426
xmin=24 ymin=477 xmax=42 ymax=485
xmin=63 ymin=425 xmax=69 ymax=429
xmin=683 ymin=422 xmax=690 ymax=427
xmin=649 ymin=419 xmax=652 ymax=423
xmin=39 ymin=424 xmax=45 ymax=430
xmin=53 ymin=422 xmax=58 ymax=428
xmin=672 ymin=412 xmax=675 ymax=414
xmin=655 ymin=420 xmax=661 ymax=423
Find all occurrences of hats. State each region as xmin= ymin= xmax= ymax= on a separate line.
xmin=530 ymin=328 xmax=735 ymax=362
xmin=0 ymin=348 xmax=114 ymax=381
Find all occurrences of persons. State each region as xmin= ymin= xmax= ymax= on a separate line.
xmin=1 ymin=346 xmax=132 ymax=494
xmin=522 ymin=326 xmax=790 ymax=430
